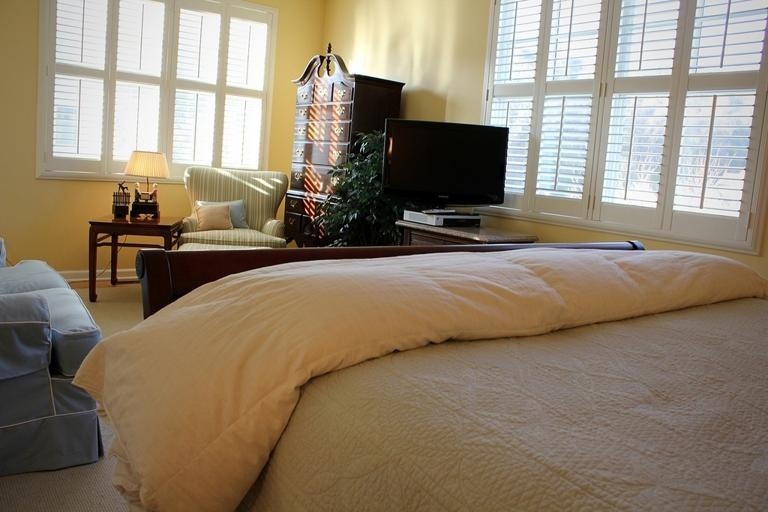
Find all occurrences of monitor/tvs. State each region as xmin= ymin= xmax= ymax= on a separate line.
xmin=382 ymin=118 xmax=509 ymax=212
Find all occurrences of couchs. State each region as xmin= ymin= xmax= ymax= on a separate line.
xmin=177 ymin=165 xmax=289 ymax=250
xmin=1 ymin=234 xmax=105 ymax=476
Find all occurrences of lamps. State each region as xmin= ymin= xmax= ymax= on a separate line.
xmin=125 ymin=149 xmax=171 ymax=221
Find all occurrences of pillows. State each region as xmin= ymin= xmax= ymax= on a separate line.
xmin=194 ymin=198 xmax=250 ymax=229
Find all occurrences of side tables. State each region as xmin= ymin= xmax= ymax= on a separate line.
xmin=90 ymin=214 xmax=183 ymax=305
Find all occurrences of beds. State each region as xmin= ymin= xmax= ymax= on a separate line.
xmin=136 ymin=240 xmax=767 ymax=511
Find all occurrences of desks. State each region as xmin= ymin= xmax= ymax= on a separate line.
xmin=396 ymin=219 xmax=537 ymax=242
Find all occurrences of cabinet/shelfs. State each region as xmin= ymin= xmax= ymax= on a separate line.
xmin=284 ymin=195 xmax=346 ymax=241
xmin=285 ymin=52 xmax=406 ymax=202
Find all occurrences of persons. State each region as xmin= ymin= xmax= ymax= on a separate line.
xmin=151 ymin=183 xmax=159 ymax=203
xmin=133 ymin=182 xmax=140 ymax=204
xmin=118 ymin=180 xmax=129 ymax=194
